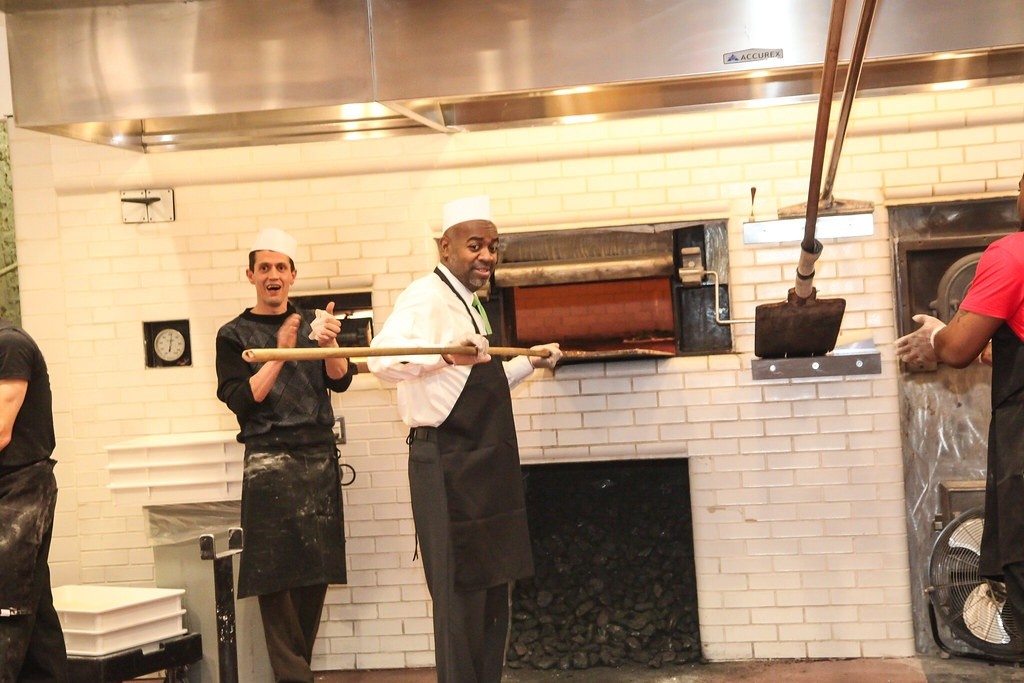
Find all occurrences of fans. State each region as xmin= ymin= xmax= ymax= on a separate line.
xmin=924 ymin=508 xmax=1024 ymax=663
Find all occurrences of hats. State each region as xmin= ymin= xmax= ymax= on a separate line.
xmin=248 ymin=229 xmax=297 ymax=271
xmin=441 ymin=196 xmax=490 ymax=235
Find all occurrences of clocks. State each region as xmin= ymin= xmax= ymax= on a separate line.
xmin=154 ymin=329 xmax=185 ymax=362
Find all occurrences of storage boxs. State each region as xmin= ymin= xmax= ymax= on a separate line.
xmin=52 ymin=585 xmax=188 ymax=656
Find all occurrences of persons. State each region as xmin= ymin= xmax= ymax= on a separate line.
xmin=365 ymin=195 xmax=564 ymax=683
xmin=1 ymin=326 xmax=68 ymax=683
xmin=215 ymin=228 xmax=354 ymax=683
xmin=891 ymin=173 xmax=1024 ymax=641
